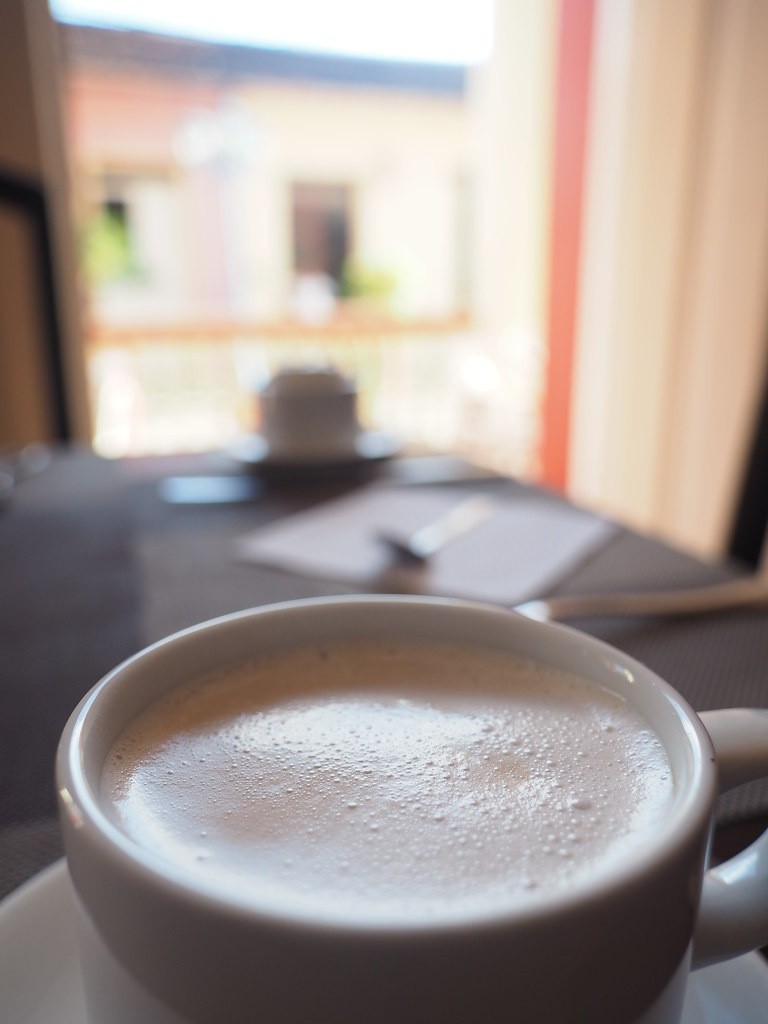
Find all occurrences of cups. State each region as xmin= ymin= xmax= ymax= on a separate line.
xmin=54 ymin=590 xmax=768 ymax=1024
xmin=260 ymin=368 xmax=361 ymax=468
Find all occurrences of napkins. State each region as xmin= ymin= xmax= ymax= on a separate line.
xmin=230 ymin=473 xmax=618 ymax=612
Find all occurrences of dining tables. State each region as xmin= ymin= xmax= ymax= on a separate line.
xmin=0 ymin=440 xmax=768 ymax=965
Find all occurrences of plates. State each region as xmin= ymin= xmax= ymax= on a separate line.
xmin=0 ymin=850 xmax=768 ymax=1024
